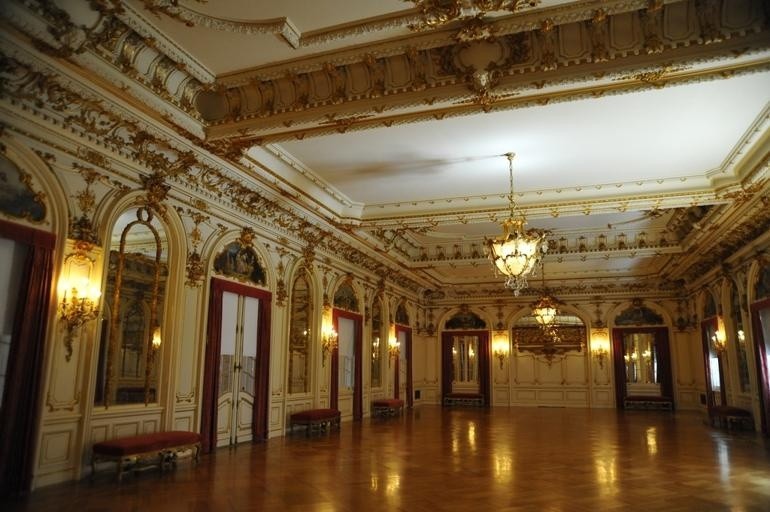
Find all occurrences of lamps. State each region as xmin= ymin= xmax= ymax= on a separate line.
xmin=708 ymin=329 xmax=729 ymax=357
xmin=531 ymin=256 xmax=561 ymax=342
xmin=488 ymin=150 xmax=549 ymax=298
xmin=322 ymin=331 xmax=340 ymax=358
xmin=56 ymin=275 xmax=105 ymax=361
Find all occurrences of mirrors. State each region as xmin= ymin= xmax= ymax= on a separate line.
xmin=449 ymin=334 xmax=480 ymax=394
xmin=625 ymin=332 xmax=661 ymax=396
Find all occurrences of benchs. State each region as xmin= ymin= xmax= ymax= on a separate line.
xmin=289 ymin=406 xmax=339 ymax=436
xmin=372 ymin=396 xmax=405 ymax=421
xmin=705 ymin=402 xmax=753 ymax=434
xmin=88 ymin=430 xmax=201 ymax=482
